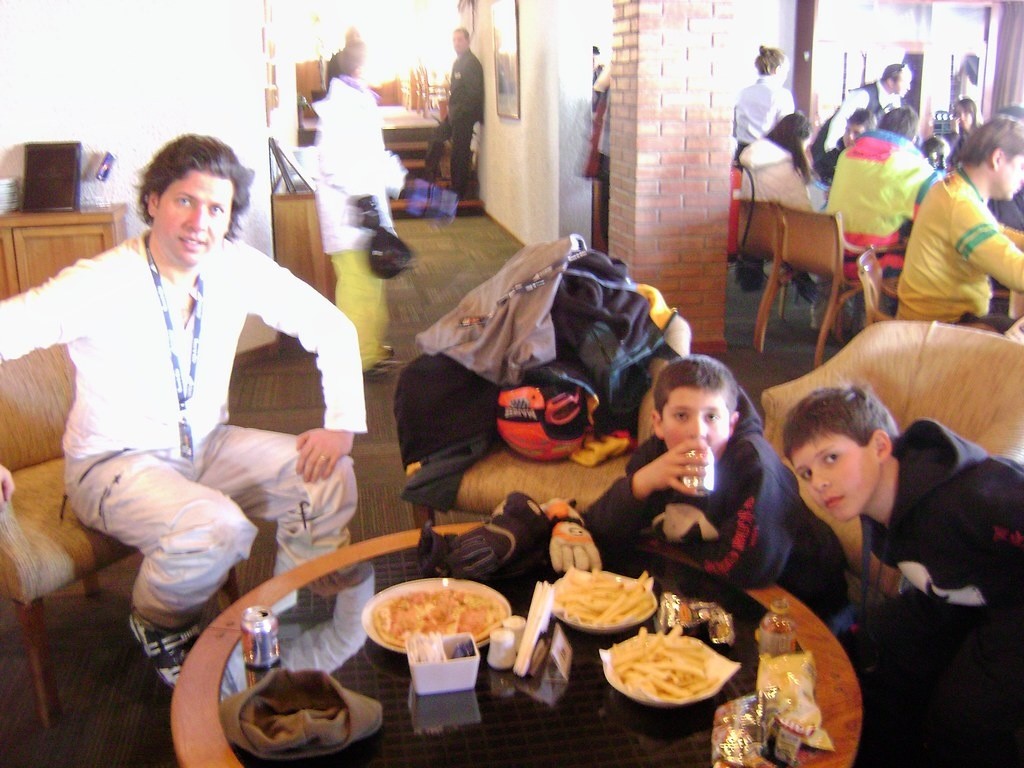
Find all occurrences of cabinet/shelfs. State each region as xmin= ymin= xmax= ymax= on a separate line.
xmin=0 ymin=204 xmax=129 ymax=304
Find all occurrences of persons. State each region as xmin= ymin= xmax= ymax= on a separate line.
xmin=542 ymin=40 xmax=1024 ymax=353
xmin=732 ymin=44 xmax=793 ymax=177
xmin=0 ymin=135 xmax=367 ymax=688
xmin=825 ymin=107 xmax=937 ymax=331
xmin=739 ymin=114 xmax=813 ymax=215
xmin=808 ymin=62 xmax=912 ymax=178
xmin=897 ymin=112 xmax=1024 ymax=322
xmin=424 ymin=28 xmax=484 ymax=201
xmin=784 ymin=387 xmax=1024 ymax=768
xmin=317 ymin=43 xmax=413 ymax=377
xmin=578 ymin=355 xmax=853 ymax=625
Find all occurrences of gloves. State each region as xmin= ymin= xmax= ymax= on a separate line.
xmin=448 ymin=490 xmax=552 ymax=582
xmin=540 ymin=498 xmax=601 ymax=573
xmin=417 ymin=521 xmax=543 ymax=584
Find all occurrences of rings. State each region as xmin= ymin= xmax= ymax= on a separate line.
xmin=318 ymin=456 xmax=330 ymax=463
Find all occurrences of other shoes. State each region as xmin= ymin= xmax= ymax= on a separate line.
xmin=363 ymin=366 xmax=391 ymax=381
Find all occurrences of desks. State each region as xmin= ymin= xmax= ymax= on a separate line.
xmin=272 ymin=168 xmax=339 ymax=306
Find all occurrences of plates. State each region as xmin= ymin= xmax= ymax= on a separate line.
xmin=0 ymin=180 xmax=19 ymax=211
xmin=362 ymin=578 xmax=511 ymax=654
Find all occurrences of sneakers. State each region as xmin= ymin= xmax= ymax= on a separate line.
xmin=130 ymin=612 xmax=203 ymax=689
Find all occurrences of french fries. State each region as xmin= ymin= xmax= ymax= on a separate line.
xmin=555 ymin=569 xmax=655 ymax=625
xmin=608 ymin=622 xmax=720 ymax=699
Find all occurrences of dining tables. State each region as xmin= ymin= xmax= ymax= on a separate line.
xmin=171 ymin=522 xmax=862 ymax=768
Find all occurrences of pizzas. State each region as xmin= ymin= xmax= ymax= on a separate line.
xmin=372 ymin=587 xmax=507 ymax=650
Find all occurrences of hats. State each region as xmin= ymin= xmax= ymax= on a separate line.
xmin=222 ymin=666 xmax=381 ymax=757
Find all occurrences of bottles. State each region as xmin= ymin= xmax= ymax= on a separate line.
xmin=760 ymin=595 xmax=794 ymax=659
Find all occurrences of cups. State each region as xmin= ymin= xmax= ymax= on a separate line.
xmin=486 ymin=615 xmax=528 ymax=669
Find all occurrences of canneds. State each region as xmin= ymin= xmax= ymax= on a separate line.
xmin=240 ymin=606 xmax=280 ymax=668
xmin=681 ymin=445 xmax=713 ymax=494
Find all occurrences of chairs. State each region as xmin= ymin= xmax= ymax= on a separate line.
xmin=405 ymin=315 xmax=692 ymax=530
xmin=0 ymin=344 xmax=239 ymax=732
xmin=736 ymin=197 xmax=1009 ymax=369
xmin=760 ymin=320 xmax=1024 ymax=597
xmin=409 ymin=64 xmax=452 ymax=119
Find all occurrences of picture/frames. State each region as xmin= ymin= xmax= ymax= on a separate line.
xmin=20 ymin=141 xmax=83 ymax=212
xmin=490 ymin=0 xmax=519 ymax=119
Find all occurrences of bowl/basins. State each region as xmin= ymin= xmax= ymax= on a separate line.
xmin=599 ymin=633 xmax=741 ymax=707
xmin=552 ymin=570 xmax=657 ymax=633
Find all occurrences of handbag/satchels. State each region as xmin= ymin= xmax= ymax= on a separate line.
xmin=370 ymin=225 xmax=410 ymax=278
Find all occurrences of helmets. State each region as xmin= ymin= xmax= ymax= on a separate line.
xmin=498 ymin=384 xmax=588 ymax=462
